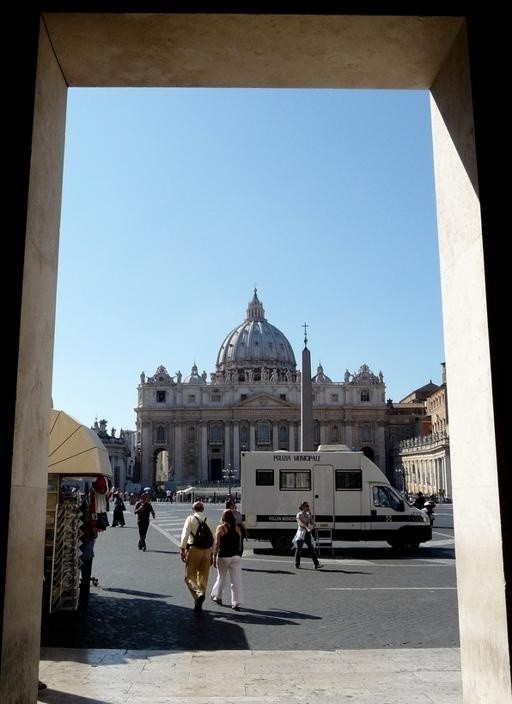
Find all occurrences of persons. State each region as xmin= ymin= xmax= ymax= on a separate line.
xmin=77 ymin=504 xmax=95 ymax=609
xmin=378 ymin=370 xmax=384 ymax=383
xmin=110 ymin=491 xmax=125 ymax=527
xmin=224 ymin=367 xmax=301 ymax=384
xmin=209 ymin=508 xmax=243 ymax=611
xmin=291 ymin=501 xmax=324 ymax=570
xmin=133 ymin=491 xmax=156 ymax=552
xmin=139 ymin=370 xmax=146 ymax=384
xmin=178 ymin=500 xmax=216 ymax=617
xmin=175 ymin=371 xmax=182 ymax=383
xmin=201 ymin=370 xmax=207 ymax=386
xmin=413 ymin=492 xmax=426 ymax=510
xmin=166 ymin=489 xmax=173 ymax=504
xmin=343 ymin=368 xmax=350 ymax=382
xmin=225 ymin=500 xmax=248 ymax=558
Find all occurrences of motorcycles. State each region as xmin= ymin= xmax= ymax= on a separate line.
xmin=409 ymin=500 xmax=436 ymax=526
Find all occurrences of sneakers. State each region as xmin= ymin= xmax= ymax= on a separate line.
xmin=295 ymin=563 xmax=300 ymax=569
xmin=194 ymin=595 xmax=206 ymax=614
xmin=315 ymin=564 xmax=324 ymax=569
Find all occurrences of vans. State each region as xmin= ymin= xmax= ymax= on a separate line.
xmin=241 ymin=444 xmax=435 ymax=556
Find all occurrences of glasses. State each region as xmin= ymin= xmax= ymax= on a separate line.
xmin=305 ymin=505 xmax=308 ymax=507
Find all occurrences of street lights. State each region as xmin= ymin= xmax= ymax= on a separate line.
xmin=395 ymin=461 xmax=408 ymax=493
xmin=222 ymin=463 xmax=237 ymax=494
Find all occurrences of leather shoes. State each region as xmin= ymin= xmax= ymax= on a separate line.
xmin=209 ymin=594 xmax=223 ymax=605
xmin=232 ymin=603 xmax=240 ymax=611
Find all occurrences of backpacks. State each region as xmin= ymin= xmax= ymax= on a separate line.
xmin=191 ymin=516 xmax=214 ymax=548
